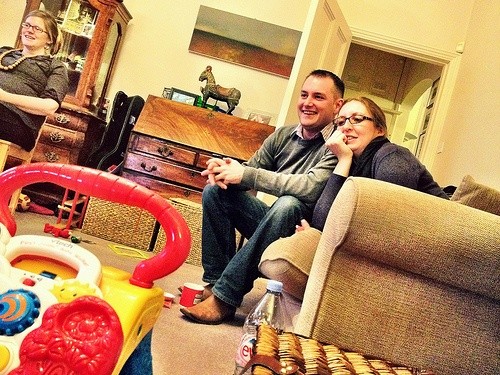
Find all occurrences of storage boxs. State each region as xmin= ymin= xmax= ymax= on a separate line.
xmin=82 ymin=196 xmax=156 ymax=250
xmin=150 ymin=197 xmax=242 ymax=268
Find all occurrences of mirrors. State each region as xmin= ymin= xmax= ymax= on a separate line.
xmin=92 ymin=22 xmax=121 ymax=105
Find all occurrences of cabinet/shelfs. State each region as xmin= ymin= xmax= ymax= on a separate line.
xmin=5 ymin=0 xmax=133 ymax=218
xmin=123 ymin=95 xmax=274 ymax=206
xmin=341 ymin=42 xmax=406 ymax=115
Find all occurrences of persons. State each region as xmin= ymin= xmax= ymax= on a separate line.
xmin=67 ymin=3 xmax=94 ymax=34
xmin=180 ymin=70 xmax=345 ymax=325
xmin=0 ymin=10 xmax=69 ymax=152
xmin=295 ymin=98 xmax=450 ymax=233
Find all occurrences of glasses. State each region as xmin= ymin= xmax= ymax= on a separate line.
xmin=332 ymin=115 xmax=375 ymax=127
xmin=22 ymin=22 xmax=49 ymax=34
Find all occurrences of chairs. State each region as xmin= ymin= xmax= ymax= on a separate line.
xmin=0 ymin=116 xmax=49 ymax=217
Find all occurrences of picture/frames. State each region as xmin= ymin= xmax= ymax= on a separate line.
xmin=426 ymin=77 xmax=440 ymax=109
xmin=419 ymin=109 xmax=433 ymax=136
xmin=168 ymin=87 xmax=198 ymax=106
xmin=187 ymin=5 xmax=303 ymax=79
xmin=246 ymin=112 xmax=273 ymax=125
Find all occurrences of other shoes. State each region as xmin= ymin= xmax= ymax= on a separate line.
xmin=178 ymin=286 xmax=212 ymax=302
xmin=180 ymin=299 xmax=236 ymax=326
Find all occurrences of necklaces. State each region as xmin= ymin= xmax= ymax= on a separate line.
xmin=0 ymin=48 xmax=36 ymax=70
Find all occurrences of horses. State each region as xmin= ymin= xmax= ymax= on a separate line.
xmin=198 ymin=65 xmax=241 ymax=115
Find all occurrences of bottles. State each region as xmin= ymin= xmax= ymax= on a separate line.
xmin=232 ymin=280 xmax=289 ymax=375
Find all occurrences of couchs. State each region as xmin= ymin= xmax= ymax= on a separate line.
xmin=257 ymin=175 xmax=500 ymax=375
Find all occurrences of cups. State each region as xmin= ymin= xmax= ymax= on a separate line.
xmin=180 ymin=283 xmax=205 ymax=307
